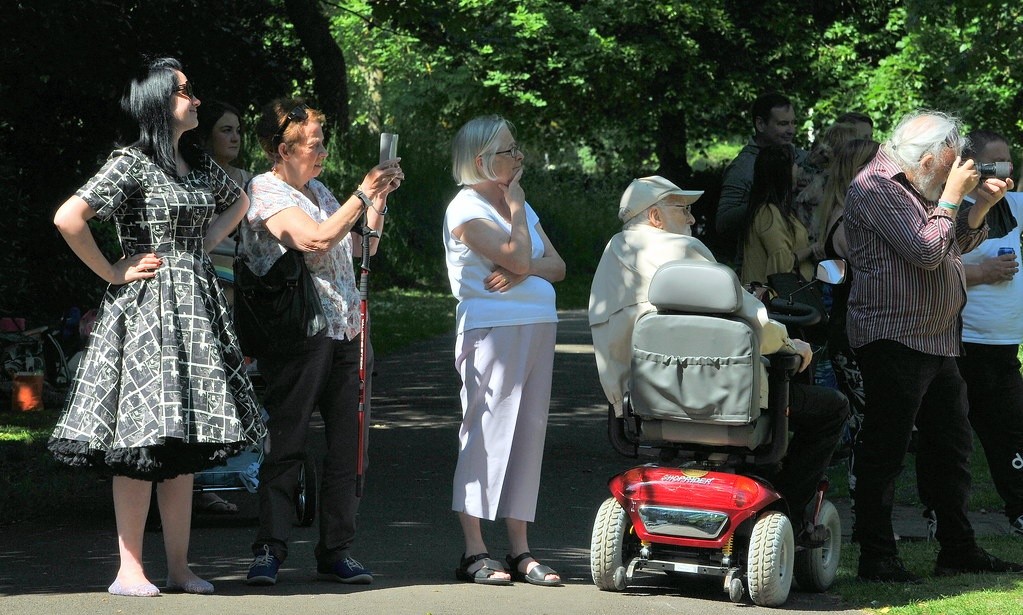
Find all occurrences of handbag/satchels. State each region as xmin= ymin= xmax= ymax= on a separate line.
xmin=232 ymin=176 xmax=328 ymax=358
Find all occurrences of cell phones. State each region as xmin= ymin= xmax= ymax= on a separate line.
xmin=380 ymin=134 xmax=398 ymax=166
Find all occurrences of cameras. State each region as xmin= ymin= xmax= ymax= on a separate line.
xmin=959 ymin=160 xmax=1014 ymax=184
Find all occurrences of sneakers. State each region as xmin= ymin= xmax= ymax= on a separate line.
xmin=246 ymin=545 xmax=279 ymax=584
xmin=317 ymin=556 xmax=374 ymax=585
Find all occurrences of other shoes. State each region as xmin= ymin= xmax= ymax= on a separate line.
xmin=933 ymin=546 xmax=1023 ymax=576
xmin=108 ymin=579 xmax=160 ymax=598
xmin=927 ymin=510 xmax=939 ymax=541
xmin=166 ymin=577 xmax=217 ymax=593
xmin=857 ymin=561 xmax=921 ymax=585
xmin=1008 ymin=516 xmax=1023 ymax=536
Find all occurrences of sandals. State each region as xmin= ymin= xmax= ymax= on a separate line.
xmin=505 ymin=552 xmax=562 ymax=586
xmin=457 ymin=552 xmax=511 ymax=585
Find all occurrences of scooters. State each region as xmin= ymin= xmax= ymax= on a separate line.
xmin=588 ymin=256 xmax=850 ymax=608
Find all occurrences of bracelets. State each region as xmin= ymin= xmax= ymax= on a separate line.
xmin=354 ymin=190 xmax=372 ymax=206
xmin=949 ymin=205 xmax=959 ymax=209
xmin=377 ymin=205 xmax=387 ymax=215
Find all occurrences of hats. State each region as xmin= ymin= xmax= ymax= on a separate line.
xmin=619 ymin=175 xmax=705 ymax=223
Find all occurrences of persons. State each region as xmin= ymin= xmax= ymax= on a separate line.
xmin=843 ymin=109 xmax=1023 ymax=583
xmin=588 ymin=175 xmax=850 ymax=549
xmin=444 ymin=114 xmax=566 ymax=586
xmin=955 ymin=131 xmax=1023 ymax=531
xmin=194 ymin=100 xmax=257 ymax=512
xmin=48 ymin=59 xmax=271 ymax=598
xmin=715 ymin=94 xmax=900 ymax=540
xmin=240 ymin=101 xmax=404 ymax=585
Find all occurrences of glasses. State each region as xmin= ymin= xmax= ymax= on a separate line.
xmin=172 ymin=81 xmax=193 ymax=99
xmin=917 ymin=123 xmax=960 ymax=163
xmin=487 ymin=145 xmax=521 ymax=159
xmin=271 ymin=102 xmax=310 ymax=147
xmin=661 ymin=203 xmax=691 ymax=216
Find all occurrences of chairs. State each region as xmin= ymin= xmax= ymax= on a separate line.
xmin=627 ymin=262 xmax=771 ymax=446
